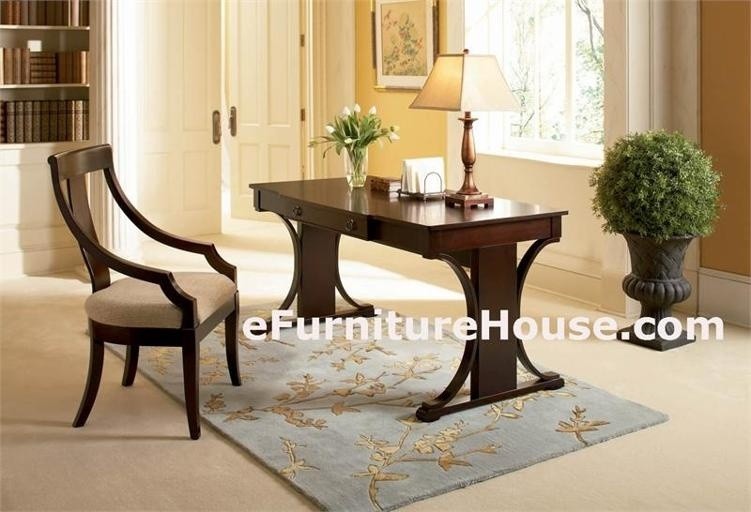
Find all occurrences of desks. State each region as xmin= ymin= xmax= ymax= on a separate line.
xmin=249 ymin=175 xmax=569 ymax=422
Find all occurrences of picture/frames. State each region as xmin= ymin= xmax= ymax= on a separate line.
xmin=371 ymin=0 xmax=438 ymax=91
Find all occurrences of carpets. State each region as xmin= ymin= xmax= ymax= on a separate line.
xmin=87 ymin=295 xmax=668 ymax=512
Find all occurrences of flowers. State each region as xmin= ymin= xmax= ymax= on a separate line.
xmin=307 ymin=104 xmax=401 ymax=183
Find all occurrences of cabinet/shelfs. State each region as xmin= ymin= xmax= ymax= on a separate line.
xmin=0 ymin=0 xmax=91 ymax=143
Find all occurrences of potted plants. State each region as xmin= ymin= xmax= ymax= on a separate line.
xmin=588 ymin=129 xmax=728 ymax=350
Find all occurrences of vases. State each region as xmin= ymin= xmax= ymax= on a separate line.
xmin=344 ymin=147 xmax=367 ymax=187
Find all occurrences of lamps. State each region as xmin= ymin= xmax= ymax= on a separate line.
xmin=408 ymin=48 xmax=522 ymax=209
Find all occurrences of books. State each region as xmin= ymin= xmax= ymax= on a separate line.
xmin=0 ymin=99 xmax=89 ymax=143
xmin=371 ymin=156 xmax=445 ymax=193
xmin=0 ymin=47 xmax=89 ymax=85
xmin=0 ymin=0 xmax=89 ymax=26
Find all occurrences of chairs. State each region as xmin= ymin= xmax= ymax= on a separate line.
xmin=47 ymin=143 xmax=241 ymax=441
